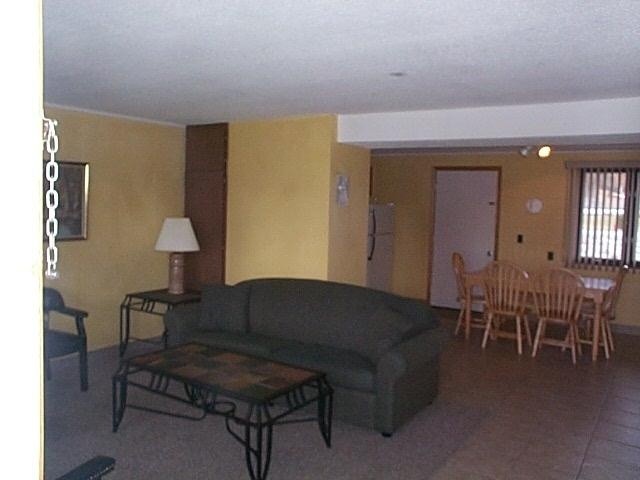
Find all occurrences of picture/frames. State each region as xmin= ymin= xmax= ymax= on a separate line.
xmin=43 ymin=160 xmax=90 ymax=241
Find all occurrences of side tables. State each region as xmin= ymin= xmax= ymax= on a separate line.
xmin=119 ymin=288 xmax=203 ymax=358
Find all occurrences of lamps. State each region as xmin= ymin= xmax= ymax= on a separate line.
xmin=154 ymin=217 xmax=200 ymax=294
xmin=521 ymin=146 xmax=552 ymax=158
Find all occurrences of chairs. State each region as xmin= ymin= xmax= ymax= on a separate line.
xmin=452 ymin=252 xmax=629 ymax=365
xmin=43 ymin=287 xmax=90 ymax=392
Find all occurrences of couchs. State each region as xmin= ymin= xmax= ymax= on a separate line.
xmin=164 ymin=277 xmax=445 ymax=437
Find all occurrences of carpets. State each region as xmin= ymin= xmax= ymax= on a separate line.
xmin=44 ymin=330 xmax=484 ymax=480
